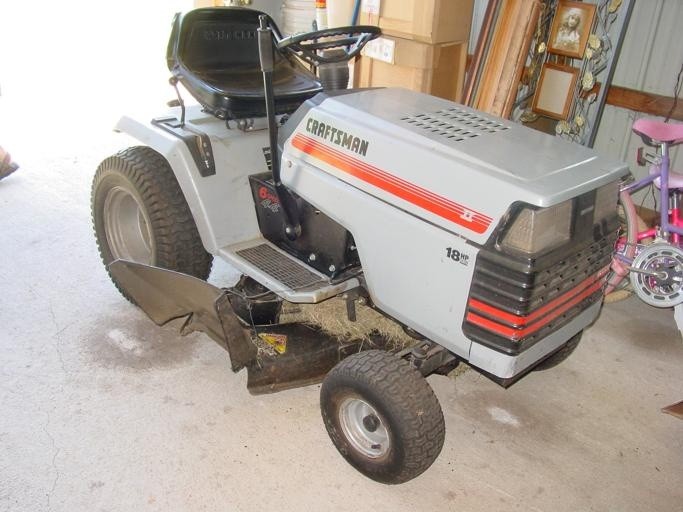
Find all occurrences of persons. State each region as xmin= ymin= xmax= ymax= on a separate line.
xmin=555 ymin=8 xmax=581 ymax=51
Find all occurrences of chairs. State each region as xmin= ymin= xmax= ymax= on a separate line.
xmin=166 ymin=6 xmax=324 ymax=121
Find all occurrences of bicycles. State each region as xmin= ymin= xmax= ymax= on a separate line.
xmin=602 ymin=115 xmax=683 ymax=333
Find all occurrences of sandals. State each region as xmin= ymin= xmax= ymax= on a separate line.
xmin=0 ymin=149 xmax=20 ymax=180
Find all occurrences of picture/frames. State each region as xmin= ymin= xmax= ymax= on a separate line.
xmin=532 ymin=62 xmax=580 ymax=121
xmin=545 ymin=0 xmax=597 ymax=60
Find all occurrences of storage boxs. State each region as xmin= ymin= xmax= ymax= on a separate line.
xmin=354 ymin=33 xmax=468 ymax=103
xmin=360 ymin=0 xmax=473 ymax=43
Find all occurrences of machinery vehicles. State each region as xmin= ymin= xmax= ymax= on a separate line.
xmin=90 ymin=6 xmax=632 ymax=484
